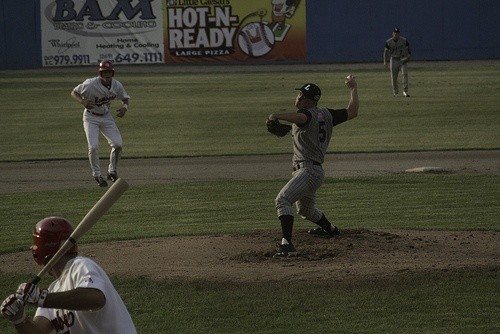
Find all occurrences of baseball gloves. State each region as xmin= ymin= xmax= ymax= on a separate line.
xmin=266 ymin=118 xmax=293 ymax=137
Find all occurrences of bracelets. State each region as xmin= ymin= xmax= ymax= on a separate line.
xmin=122 ymin=104 xmax=127 ymax=109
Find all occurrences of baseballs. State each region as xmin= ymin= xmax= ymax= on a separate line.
xmin=346 ymin=75 xmax=356 ymax=84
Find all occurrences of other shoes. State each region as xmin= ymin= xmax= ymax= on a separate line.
xmin=108 ymin=172 xmax=118 ymax=181
xmin=307 ymin=226 xmax=340 ymax=235
xmin=95 ymin=176 xmax=108 ymax=187
xmin=272 ymin=244 xmax=296 ymax=258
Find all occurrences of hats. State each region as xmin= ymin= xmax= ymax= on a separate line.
xmin=295 ymin=83 xmax=321 ymax=102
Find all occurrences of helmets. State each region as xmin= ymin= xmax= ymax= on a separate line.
xmin=98 ymin=60 xmax=114 ymax=71
xmin=33 ymin=217 xmax=78 ymax=265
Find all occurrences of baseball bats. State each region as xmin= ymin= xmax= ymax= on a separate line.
xmin=17 ymin=177 xmax=130 ymax=304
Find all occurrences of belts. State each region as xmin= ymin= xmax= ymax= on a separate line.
xmin=293 ymin=162 xmax=321 ymax=171
xmin=87 ymin=110 xmax=104 ymax=116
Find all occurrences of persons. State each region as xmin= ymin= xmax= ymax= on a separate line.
xmin=263 ymin=75 xmax=359 ymax=258
xmin=0 ymin=217 xmax=138 ymax=334
xmin=70 ymin=60 xmax=130 ymax=187
xmin=383 ymin=28 xmax=411 ymax=97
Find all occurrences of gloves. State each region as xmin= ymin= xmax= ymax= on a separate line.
xmin=16 ymin=283 xmax=47 ymax=308
xmin=0 ymin=294 xmax=26 ymax=323
